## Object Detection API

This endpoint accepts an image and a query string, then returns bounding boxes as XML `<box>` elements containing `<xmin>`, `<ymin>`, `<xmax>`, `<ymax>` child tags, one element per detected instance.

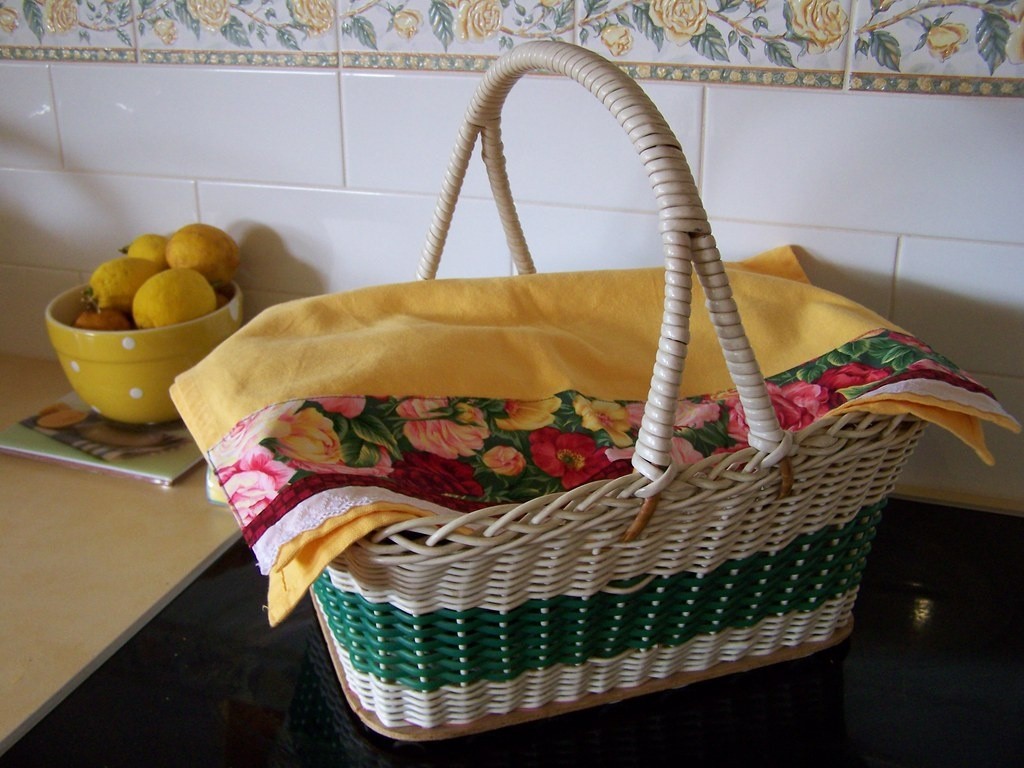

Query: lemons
<box><xmin>71</xmin><ymin>222</ymin><xmax>238</xmax><ymax>331</ymax></box>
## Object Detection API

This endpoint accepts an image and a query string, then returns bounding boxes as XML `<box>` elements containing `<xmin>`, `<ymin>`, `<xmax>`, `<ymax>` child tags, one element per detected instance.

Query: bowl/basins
<box><xmin>43</xmin><ymin>276</ymin><xmax>243</xmax><ymax>425</ymax></box>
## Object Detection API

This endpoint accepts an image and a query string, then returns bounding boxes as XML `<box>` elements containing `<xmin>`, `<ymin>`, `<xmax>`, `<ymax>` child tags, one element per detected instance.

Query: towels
<box><xmin>165</xmin><ymin>235</ymin><xmax>1024</xmax><ymax>629</ymax></box>
